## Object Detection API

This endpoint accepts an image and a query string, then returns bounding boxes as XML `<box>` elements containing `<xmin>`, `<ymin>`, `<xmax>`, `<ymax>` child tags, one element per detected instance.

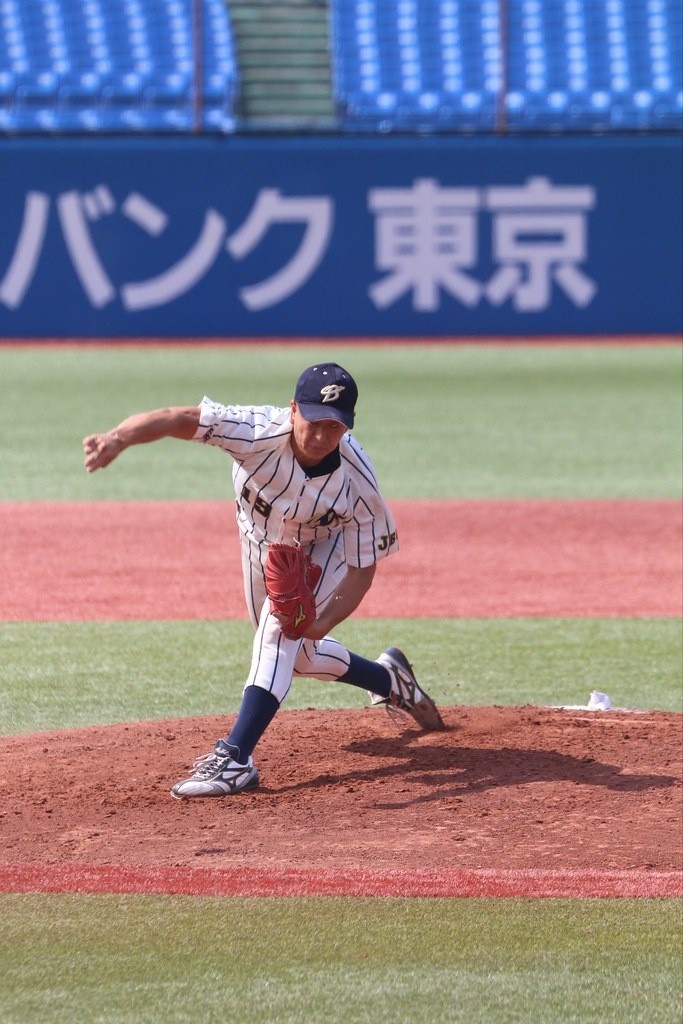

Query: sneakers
<box><xmin>367</xmin><ymin>647</ymin><xmax>444</xmax><ymax>732</ymax></box>
<box><xmin>170</xmin><ymin>738</ymin><xmax>259</xmax><ymax>800</ymax></box>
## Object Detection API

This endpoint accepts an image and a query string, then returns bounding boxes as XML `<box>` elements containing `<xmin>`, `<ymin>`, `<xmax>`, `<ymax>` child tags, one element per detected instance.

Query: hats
<box><xmin>294</xmin><ymin>363</ymin><xmax>358</xmax><ymax>430</ymax></box>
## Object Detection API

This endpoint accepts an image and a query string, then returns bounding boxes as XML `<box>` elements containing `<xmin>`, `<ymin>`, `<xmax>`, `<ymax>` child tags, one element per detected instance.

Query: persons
<box><xmin>83</xmin><ymin>362</ymin><xmax>444</xmax><ymax>799</ymax></box>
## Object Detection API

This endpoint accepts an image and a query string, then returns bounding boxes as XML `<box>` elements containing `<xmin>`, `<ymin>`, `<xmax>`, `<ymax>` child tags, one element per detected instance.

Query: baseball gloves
<box><xmin>262</xmin><ymin>539</ymin><xmax>324</xmax><ymax>642</ymax></box>
